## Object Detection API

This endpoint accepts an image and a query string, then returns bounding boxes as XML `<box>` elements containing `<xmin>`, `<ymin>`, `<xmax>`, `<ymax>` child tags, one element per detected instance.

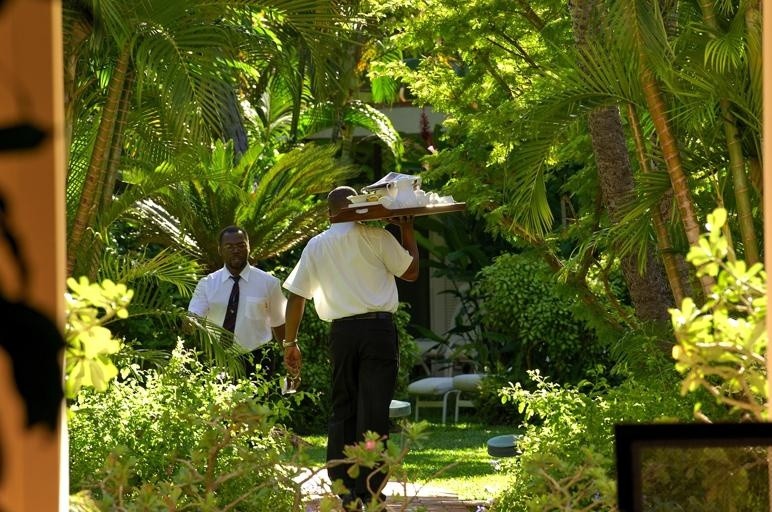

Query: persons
<box><xmin>280</xmin><ymin>184</ymin><xmax>422</xmax><ymax>512</ymax></box>
<box><xmin>186</xmin><ymin>223</ymin><xmax>288</xmax><ymax>381</ymax></box>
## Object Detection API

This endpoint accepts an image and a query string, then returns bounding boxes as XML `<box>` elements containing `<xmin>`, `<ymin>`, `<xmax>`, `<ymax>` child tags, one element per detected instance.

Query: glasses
<box><xmin>221</xmin><ymin>242</ymin><xmax>248</xmax><ymax>253</ymax></box>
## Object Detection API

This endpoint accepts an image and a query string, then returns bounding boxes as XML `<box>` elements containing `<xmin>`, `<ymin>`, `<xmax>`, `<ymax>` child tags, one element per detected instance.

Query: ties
<box><xmin>219</xmin><ymin>276</ymin><xmax>242</xmax><ymax>350</ymax></box>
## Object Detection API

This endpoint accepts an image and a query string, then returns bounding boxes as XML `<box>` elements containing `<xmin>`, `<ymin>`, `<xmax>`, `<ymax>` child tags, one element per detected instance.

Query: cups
<box><xmin>429</xmin><ymin>193</ymin><xmax>454</xmax><ymax>206</ymax></box>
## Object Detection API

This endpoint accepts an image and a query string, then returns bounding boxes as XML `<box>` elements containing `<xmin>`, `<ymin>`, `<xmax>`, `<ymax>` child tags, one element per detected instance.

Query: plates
<box><xmin>346</xmin><ymin>195</ymin><xmax>369</xmax><ymax>204</ymax></box>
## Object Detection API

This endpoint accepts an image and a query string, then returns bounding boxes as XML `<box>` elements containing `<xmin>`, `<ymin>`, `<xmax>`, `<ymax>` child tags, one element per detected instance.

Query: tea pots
<box><xmin>386</xmin><ymin>177</ymin><xmax>433</xmax><ymax>213</ymax></box>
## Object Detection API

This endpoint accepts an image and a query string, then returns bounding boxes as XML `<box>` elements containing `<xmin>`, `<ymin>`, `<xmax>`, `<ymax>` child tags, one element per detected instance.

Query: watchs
<box><xmin>282</xmin><ymin>337</ymin><xmax>299</xmax><ymax>350</ymax></box>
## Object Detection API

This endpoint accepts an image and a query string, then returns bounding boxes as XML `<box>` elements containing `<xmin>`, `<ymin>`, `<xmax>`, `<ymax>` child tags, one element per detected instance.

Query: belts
<box><xmin>331</xmin><ymin>311</ymin><xmax>394</xmax><ymax>321</ymax></box>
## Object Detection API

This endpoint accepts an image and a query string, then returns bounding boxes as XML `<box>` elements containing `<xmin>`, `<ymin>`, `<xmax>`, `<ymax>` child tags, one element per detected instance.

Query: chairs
<box><xmin>407</xmin><ymin>343</ymin><xmax>502</xmax><ymax>427</ymax></box>
